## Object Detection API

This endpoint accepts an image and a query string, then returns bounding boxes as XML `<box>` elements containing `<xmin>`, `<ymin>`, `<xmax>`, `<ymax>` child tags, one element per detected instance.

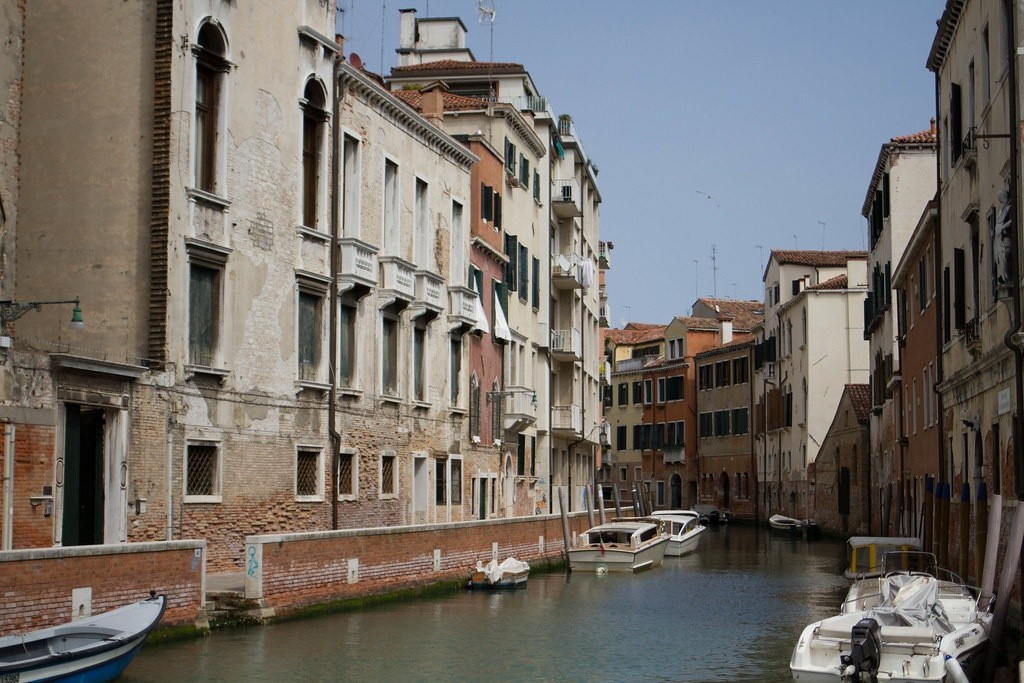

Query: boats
<box><xmin>645</xmin><ymin>510</ymin><xmax>707</xmax><ymax>557</ymax></box>
<box><xmin>2</xmin><ymin>590</ymin><xmax>171</xmax><ymax>683</ymax></box>
<box><xmin>471</xmin><ymin>557</ymin><xmax>531</xmax><ymax>588</ymax></box>
<box><xmin>790</xmin><ymin>536</ymin><xmax>992</xmax><ymax>683</ymax></box>
<box><xmin>566</xmin><ymin>516</ymin><xmax>673</xmax><ymax>576</ymax></box>
<box><xmin>769</xmin><ymin>513</ymin><xmax>803</xmax><ymax>532</ymax></box>
